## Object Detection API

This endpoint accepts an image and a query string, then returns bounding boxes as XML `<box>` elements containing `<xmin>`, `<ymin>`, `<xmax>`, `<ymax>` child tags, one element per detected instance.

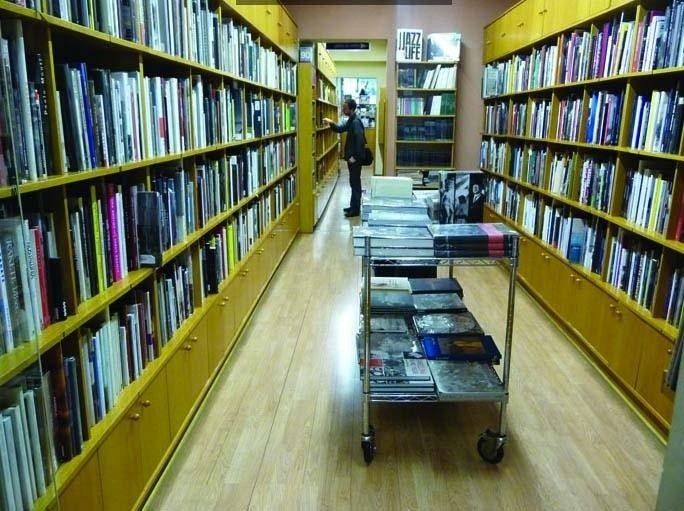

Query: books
<box><xmin>1</xmin><ymin>1</ymin><xmax>297</xmax><ymax>510</ymax></box>
<box><xmin>343</xmin><ymin>173</ymin><xmax>519</xmax><ymax>404</ymax></box>
<box><xmin>482</xmin><ymin>0</ymin><xmax>684</xmax><ymax>328</ymax></box>
<box><xmin>396</xmin><ymin>65</ymin><xmax>456</xmax><ymax>185</ymax></box>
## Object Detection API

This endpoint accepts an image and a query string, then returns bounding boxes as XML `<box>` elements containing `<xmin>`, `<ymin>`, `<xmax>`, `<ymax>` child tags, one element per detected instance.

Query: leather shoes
<box><xmin>344</xmin><ymin>207</ymin><xmax>359</xmax><ymax>217</ymax></box>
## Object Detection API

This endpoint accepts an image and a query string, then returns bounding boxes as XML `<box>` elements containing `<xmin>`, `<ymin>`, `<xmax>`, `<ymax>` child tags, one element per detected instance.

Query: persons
<box><xmin>322</xmin><ymin>99</ymin><xmax>365</xmax><ymax>218</ymax></box>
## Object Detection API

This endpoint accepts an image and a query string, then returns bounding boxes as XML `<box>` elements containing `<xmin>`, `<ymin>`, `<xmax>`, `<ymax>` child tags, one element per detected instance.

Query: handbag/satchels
<box><xmin>361</xmin><ymin>148</ymin><xmax>373</xmax><ymax>165</ymax></box>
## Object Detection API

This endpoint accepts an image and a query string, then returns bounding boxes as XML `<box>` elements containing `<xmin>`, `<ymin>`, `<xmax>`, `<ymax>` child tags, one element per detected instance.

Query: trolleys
<box><xmin>360</xmin><ymin>184</ymin><xmax>518</xmax><ymax>466</ymax></box>
